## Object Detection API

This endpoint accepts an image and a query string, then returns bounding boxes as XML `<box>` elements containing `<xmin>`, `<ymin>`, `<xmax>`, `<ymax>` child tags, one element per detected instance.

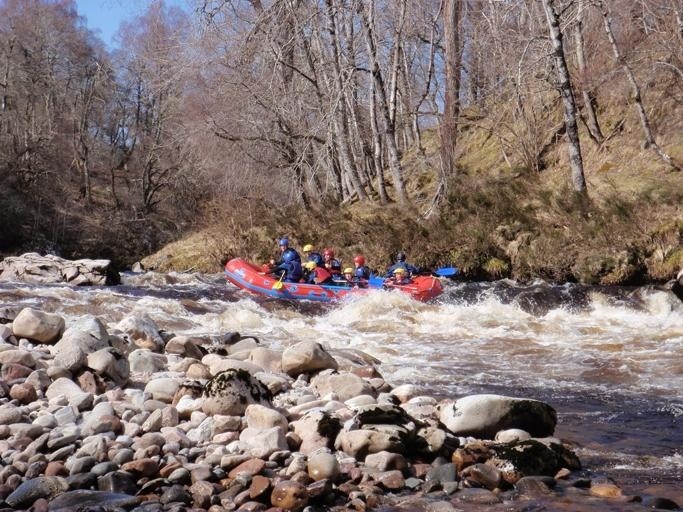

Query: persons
<box><xmin>300</xmin><ymin>243</ymin><xmax>370</xmax><ymax>292</ymax></box>
<box><xmin>262</xmin><ymin>237</ymin><xmax>301</xmax><ymax>269</ymax></box>
<box><xmin>254</xmin><ymin>248</ymin><xmax>302</xmax><ymax>283</ymax></box>
<box><xmin>383</xmin><ymin>268</ymin><xmax>414</xmax><ymax>288</ymax></box>
<box><xmin>384</xmin><ymin>252</ymin><xmax>420</xmax><ymax>279</ymax></box>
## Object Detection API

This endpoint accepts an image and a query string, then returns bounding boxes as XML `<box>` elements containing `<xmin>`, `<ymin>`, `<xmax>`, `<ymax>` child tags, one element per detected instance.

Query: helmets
<box><xmin>394</xmin><ymin>268</ymin><xmax>404</xmax><ymax>276</ymax></box>
<box><xmin>353</xmin><ymin>255</ymin><xmax>365</xmax><ymax>266</ymax></box>
<box><xmin>343</xmin><ymin>268</ymin><xmax>355</xmax><ymax>277</ymax></box>
<box><xmin>278</xmin><ymin>239</ymin><xmax>289</xmax><ymax>248</ymax></box>
<box><xmin>397</xmin><ymin>253</ymin><xmax>406</xmax><ymax>261</ymax></box>
<box><xmin>305</xmin><ymin>261</ymin><xmax>317</xmax><ymax>272</ymax></box>
<box><xmin>302</xmin><ymin>244</ymin><xmax>316</xmax><ymax>253</ymax></box>
<box><xmin>323</xmin><ymin>249</ymin><xmax>335</xmax><ymax>259</ymax></box>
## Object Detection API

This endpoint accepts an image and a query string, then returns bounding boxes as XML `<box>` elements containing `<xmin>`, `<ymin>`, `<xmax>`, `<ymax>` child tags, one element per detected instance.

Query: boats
<box><xmin>224</xmin><ymin>257</ymin><xmax>444</xmax><ymax>305</ymax></box>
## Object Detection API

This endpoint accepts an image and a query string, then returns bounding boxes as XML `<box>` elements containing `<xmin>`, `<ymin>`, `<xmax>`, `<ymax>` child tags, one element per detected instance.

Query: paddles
<box><xmin>332</xmin><ymin>278</ymin><xmax>384</xmax><ymax>288</ymax></box>
<box><xmin>369</xmin><ymin>270</ymin><xmax>374</xmax><ymax>278</ymax></box>
<box><xmin>412</xmin><ymin>268</ymin><xmax>458</xmax><ymax>275</ymax></box>
<box><xmin>271</xmin><ymin>270</ymin><xmax>285</xmax><ymax>289</ymax></box>
